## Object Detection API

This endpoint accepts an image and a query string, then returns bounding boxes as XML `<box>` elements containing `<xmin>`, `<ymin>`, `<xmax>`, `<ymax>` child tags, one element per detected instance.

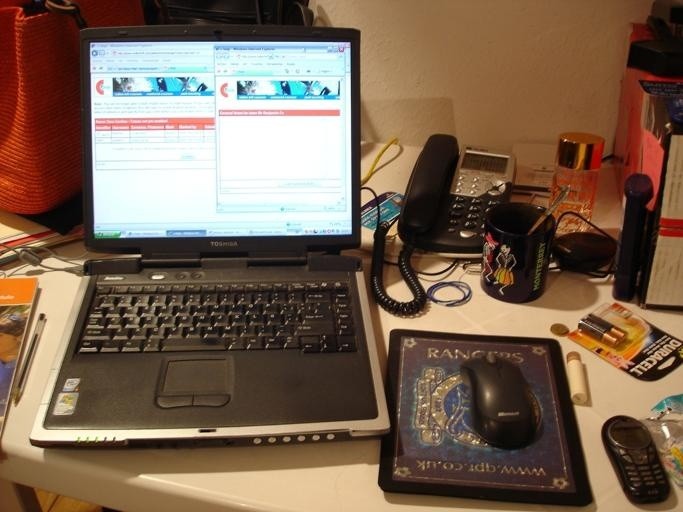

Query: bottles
<box><xmin>550</xmin><ymin>132</ymin><xmax>606</xmax><ymax>234</ymax></box>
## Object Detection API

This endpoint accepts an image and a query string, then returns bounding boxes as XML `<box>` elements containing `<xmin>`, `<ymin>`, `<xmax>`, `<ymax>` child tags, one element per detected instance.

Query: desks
<box><xmin>0</xmin><ymin>141</ymin><xmax>683</xmax><ymax>511</ymax></box>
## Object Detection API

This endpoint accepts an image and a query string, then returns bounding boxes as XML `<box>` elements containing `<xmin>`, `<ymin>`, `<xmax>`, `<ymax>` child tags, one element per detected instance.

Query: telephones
<box><xmin>370</xmin><ymin>131</ymin><xmax>517</xmax><ymax>315</ymax></box>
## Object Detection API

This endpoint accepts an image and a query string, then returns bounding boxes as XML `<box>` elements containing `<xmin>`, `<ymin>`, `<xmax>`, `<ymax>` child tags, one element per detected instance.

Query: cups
<box><xmin>480</xmin><ymin>202</ymin><xmax>556</xmax><ymax>303</ymax></box>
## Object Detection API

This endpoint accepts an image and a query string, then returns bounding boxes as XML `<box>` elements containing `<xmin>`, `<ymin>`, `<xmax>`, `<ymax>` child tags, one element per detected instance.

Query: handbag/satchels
<box><xmin>0</xmin><ymin>0</ymin><xmax>148</xmax><ymax>217</ymax></box>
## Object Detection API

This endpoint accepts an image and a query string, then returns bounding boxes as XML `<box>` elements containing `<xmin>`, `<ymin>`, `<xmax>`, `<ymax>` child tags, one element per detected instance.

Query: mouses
<box><xmin>459</xmin><ymin>352</ymin><xmax>535</xmax><ymax>450</ymax></box>
<box><xmin>553</xmin><ymin>232</ymin><xmax>616</xmax><ymax>273</ymax></box>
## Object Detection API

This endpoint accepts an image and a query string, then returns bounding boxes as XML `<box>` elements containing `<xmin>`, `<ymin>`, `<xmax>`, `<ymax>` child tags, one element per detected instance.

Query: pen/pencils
<box><xmin>528</xmin><ymin>184</ymin><xmax>571</xmax><ymax>234</ymax></box>
<box><xmin>13</xmin><ymin>312</ymin><xmax>45</xmax><ymax>407</ymax></box>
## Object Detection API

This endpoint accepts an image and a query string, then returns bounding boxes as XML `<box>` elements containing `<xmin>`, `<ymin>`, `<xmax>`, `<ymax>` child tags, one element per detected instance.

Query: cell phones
<box><xmin>601</xmin><ymin>415</ymin><xmax>671</xmax><ymax>505</ymax></box>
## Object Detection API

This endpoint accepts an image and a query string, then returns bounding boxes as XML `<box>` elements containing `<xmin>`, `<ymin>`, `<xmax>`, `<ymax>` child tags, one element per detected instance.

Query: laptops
<box><xmin>27</xmin><ymin>25</ymin><xmax>392</xmax><ymax>451</ymax></box>
